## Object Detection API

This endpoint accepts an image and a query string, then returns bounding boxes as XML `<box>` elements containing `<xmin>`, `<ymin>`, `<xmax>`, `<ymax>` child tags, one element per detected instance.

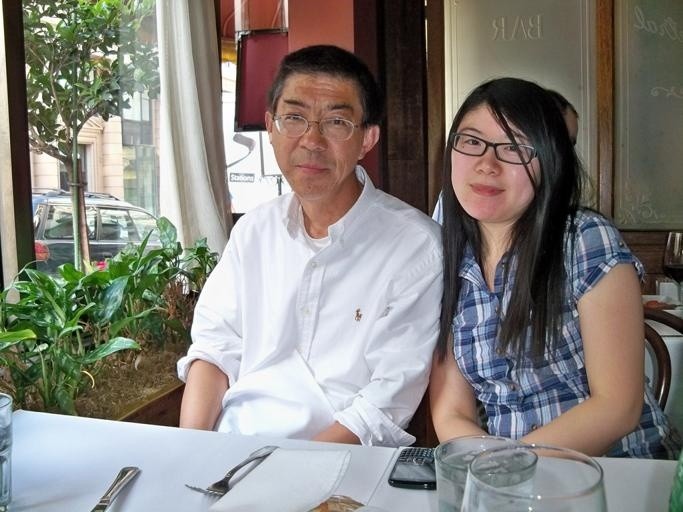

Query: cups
<box><xmin>0</xmin><ymin>393</ymin><xmax>14</xmax><ymax>512</ymax></box>
<box><xmin>434</xmin><ymin>434</ymin><xmax>607</xmax><ymax>512</ymax></box>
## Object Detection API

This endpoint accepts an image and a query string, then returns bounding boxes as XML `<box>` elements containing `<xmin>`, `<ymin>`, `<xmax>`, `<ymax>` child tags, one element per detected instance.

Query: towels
<box><xmin>209</xmin><ymin>447</ymin><xmax>353</xmax><ymax>511</ymax></box>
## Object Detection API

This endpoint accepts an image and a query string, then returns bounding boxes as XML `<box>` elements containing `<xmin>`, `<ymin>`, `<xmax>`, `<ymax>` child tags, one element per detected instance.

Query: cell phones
<box><xmin>388</xmin><ymin>447</ymin><xmax>436</xmax><ymax>490</ymax></box>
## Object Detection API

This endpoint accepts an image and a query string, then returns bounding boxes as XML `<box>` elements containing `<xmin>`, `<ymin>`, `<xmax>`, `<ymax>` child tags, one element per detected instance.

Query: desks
<box><xmin>641</xmin><ymin>290</ymin><xmax>682</xmax><ymax>433</ymax></box>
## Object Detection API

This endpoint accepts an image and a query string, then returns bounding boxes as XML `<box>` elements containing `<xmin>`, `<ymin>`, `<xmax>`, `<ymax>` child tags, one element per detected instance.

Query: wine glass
<box><xmin>662</xmin><ymin>231</ymin><xmax>683</xmax><ymax>310</ymax></box>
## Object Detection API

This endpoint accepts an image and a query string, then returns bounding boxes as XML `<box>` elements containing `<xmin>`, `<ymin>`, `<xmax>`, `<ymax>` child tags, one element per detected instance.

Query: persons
<box><xmin>427</xmin><ymin>77</ymin><xmax>681</xmax><ymax>462</ymax></box>
<box><xmin>174</xmin><ymin>43</ymin><xmax>446</xmax><ymax>447</ymax></box>
<box><xmin>431</xmin><ymin>90</ymin><xmax>579</xmax><ymax>226</ymax></box>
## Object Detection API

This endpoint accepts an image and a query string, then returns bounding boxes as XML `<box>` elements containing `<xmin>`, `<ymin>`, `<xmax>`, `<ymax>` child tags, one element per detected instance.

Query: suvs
<box><xmin>31</xmin><ymin>185</ymin><xmax>169</xmax><ymax>279</ymax></box>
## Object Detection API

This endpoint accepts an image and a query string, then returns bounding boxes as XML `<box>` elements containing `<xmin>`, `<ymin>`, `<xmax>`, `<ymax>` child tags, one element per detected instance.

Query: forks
<box><xmin>183</xmin><ymin>443</ymin><xmax>281</xmax><ymax>499</ymax></box>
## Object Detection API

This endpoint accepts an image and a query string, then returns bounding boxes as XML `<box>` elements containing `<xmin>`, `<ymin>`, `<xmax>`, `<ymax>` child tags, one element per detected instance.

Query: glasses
<box><xmin>448</xmin><ymin>132</ymin><xmax>542</xmax><ymax>165</ymax></box>
<box><xmin>272</xmin><ymin>114</ymin><xmax>368</xmax><ymax>141</ymax></box>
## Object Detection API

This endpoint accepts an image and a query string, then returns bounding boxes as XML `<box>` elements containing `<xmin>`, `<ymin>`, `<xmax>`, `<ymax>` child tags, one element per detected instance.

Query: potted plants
<box><xmin>0</xmin><ymin>217</ymin><xmax>219</xmax><ymax>410</ymax></box>
